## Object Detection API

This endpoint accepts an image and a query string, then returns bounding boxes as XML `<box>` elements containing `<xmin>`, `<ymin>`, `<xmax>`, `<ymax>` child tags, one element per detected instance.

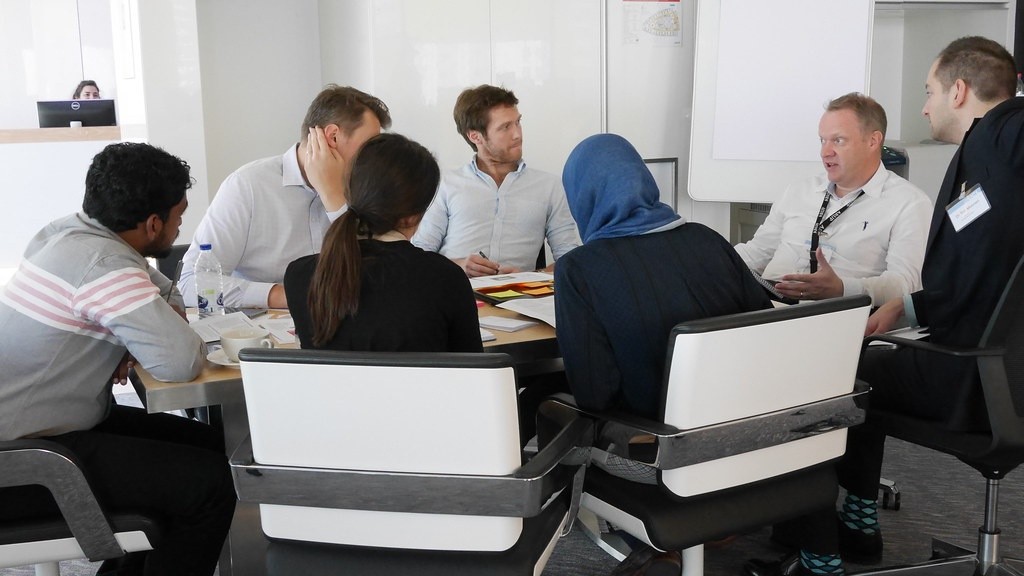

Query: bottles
<box><xmin>194</xmin><ymin>243</ymin><xmax>225</xmax><ymax>320</ymax></box>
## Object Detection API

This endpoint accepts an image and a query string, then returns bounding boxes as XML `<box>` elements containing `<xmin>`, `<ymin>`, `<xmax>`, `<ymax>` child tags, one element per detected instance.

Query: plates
<box><xmin>206</xmin><ymin>346</ymin><xmax>279</xmax><ymax>369</ymax></box>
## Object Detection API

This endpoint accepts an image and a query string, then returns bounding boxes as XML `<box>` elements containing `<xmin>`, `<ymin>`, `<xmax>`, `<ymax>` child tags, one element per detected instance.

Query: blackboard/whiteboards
<box><xmin>687</xmin><ymin>0</ymin><xmax>874</xmax><ymax>204</ymax></box>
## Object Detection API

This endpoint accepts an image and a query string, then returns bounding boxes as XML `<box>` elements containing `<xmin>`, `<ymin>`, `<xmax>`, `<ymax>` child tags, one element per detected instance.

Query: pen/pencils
<box><xmin>167</xmin><ymin>260</ymin><xmax>184</xmax><ymax>305</ymax></box>
<box><xmin>479</xmin><ymin>251</ymin><xmax>499</xmax><ymax>272</ymax></box>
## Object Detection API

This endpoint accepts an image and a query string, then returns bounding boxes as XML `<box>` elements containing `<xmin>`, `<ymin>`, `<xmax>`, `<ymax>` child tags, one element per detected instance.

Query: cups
<box><xmin>220</xmin><ymin>328</ymin><xmax>275</xmax><ymax>363</ymax></box>
<box><xmin>70</xmin><ymin>121</ymin><xmax>82</xmax><ymax>127</ymax></box>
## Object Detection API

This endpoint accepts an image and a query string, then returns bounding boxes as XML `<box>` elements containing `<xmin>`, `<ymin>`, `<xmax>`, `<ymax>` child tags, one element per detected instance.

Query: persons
<box><xmin>553</xmin><ymin>133</ymin><xmax>774</xmax><ymax>576</ymax></box>
<box><xmin>73</xmin><ymin>80</ymin><xmax>99</xmax><ymax>100</ymax></box>
<box><xmin>410</xmin><ymin>84</ymin><xmax>579</xmax><ymax>279</ymax></box>
<box><xmin>734</xmin><ymin>92</ymin><xmax>935</xmax><ymax>306</ymax></box>
<box><xmin>0</xmin><ymin>143</ymin><xmax>236</xmax><ymax>576</ymax></box>
<box><xmin>743</xmin><ymin>35</ymin><xmax>1024</xmax><ymax>575</ymax></box>
<box><xmin>283</xmin><ymin>132</ymin><xmax>558</xmax><ymax>451</ymax></box>
<box><xmin>175</xmin><ymin>86</ymin><xmax>391</xmax><ymax>308</ymax></box>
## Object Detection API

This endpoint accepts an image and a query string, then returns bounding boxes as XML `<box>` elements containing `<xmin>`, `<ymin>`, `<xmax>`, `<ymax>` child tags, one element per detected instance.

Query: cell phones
<box><xmin>236</xmin><ymin>307</ymin><xmax>268</xmax><ymax>319</ymax></box>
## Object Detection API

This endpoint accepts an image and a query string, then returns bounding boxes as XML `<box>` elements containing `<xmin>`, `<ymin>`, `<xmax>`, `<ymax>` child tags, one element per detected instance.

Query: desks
<box><xmin>128</xmin><ymin>270</ymin><xmax>555</xmax><ymax>576</ymax></box>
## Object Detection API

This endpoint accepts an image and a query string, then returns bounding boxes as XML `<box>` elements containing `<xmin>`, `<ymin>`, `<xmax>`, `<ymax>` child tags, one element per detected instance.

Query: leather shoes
<box><xmin>838</xmin><ymin>517</ymin><xmax>883</xmax><ymax>566</ymax></box>
<box><xmin>744</xmin><ymin>553</ymin><xmax>817</xmax><ymax>576</ymax></box>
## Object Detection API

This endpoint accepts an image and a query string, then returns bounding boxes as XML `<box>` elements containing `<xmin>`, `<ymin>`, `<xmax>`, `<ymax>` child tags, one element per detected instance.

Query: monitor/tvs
<box><xmin>37</xmin><ymin>99</ymin><xmax>117</xmax><ymax>127</ymax></box>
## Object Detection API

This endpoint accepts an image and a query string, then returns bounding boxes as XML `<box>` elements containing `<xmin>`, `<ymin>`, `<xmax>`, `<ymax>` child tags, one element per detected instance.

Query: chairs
<box><xmin>536</xmin><ymin>294</ymin><xmax>872</xmax><ymax>576</ymax></box>
<box><xmin>0</xmin><ymin>438</ymin><xmax>160</xmax><ymax>576</ymax></box>
<box><xmin>851</xmin><ymin>254</ymin><xmax>1024</xmax><ymax>576</ymax></box>
<box><xmin>229</xmin><ymin>347</ymin><xmax>597</xmax><ymax>575</ymax></box>
